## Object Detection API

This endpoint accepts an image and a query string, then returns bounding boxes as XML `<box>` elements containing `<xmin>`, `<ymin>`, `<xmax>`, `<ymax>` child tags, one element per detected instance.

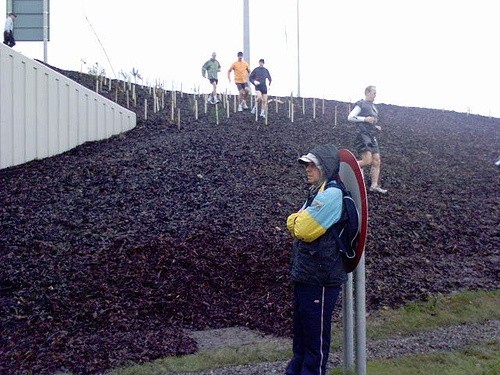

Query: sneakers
<box><xmin>369</xmin><ymin>185</ymin><xmax>388</xmax><ymax>194</ymax></box>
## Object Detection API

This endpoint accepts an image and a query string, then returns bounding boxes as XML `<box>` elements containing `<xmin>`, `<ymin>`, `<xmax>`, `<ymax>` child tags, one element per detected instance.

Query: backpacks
<box><xmin>323</xmin><ymin>182</ymin><xmax>362</xmax><ymax>263</ymax></box>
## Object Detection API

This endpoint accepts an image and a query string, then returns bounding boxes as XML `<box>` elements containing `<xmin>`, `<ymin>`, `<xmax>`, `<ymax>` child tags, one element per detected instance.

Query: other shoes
<box><xmin>214</xmin><ymin>99</ymin><xmax>220</xmax><ymax>105</ymax></box>
<box><xmin>260</xmin><ymin>113</ymin><xmax>266</xmax><ymax>118</ymax></box>
<box><xmin>237</xmin><ymin>104</ymin><xmax>243</xmax><ymax>112</ymax></box>
<box><xmin>251</xmin><ymin>106</ymin><xmax>256</xmax><ymax>114</ymax></box>
<box><xmin>242</xmin><ymin>104</ymin><xmax>249</xmax><ymax>109</ymax></box>
<box><xmin>208</xmin><ymin>98</ymin><xmax>214</xmax><ymax>105</ymax></box>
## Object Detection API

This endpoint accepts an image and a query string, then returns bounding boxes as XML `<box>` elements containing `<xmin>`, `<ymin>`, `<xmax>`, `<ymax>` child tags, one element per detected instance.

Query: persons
<box><xmin>348</xmin><ymin>85</ymin><xmax>387</xmax><ymax>194</ymax></box>
<box><xmin>3</xmin><ymin>13</ymin><xmax>16</xmax><ymax>47</ymax></box>
<box><xmin>202</xmin><ymin>53</ymin><xmax>221</xmax><ymax>105</ymax></box>
<box><xmin>249</xmin><ymin>58</ymin><xmax>272</xmax><ymax>117</ymax></box>
<box><xmin>284</xmin><ymin>143</ymin><xmax>348</xmax><ymax>375</ymax></box>
<box><xmin>227</xmin><ymin>51</ymin><xmax>251</xmax><ymax>112</ymax></box>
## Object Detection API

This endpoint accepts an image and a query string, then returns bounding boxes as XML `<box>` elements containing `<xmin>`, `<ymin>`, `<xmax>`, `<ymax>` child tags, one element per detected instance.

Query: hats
<box><xmin>297</xmin><ymin>156</ymin><xmax>313</xmax><ymax>165</ymax></box>
